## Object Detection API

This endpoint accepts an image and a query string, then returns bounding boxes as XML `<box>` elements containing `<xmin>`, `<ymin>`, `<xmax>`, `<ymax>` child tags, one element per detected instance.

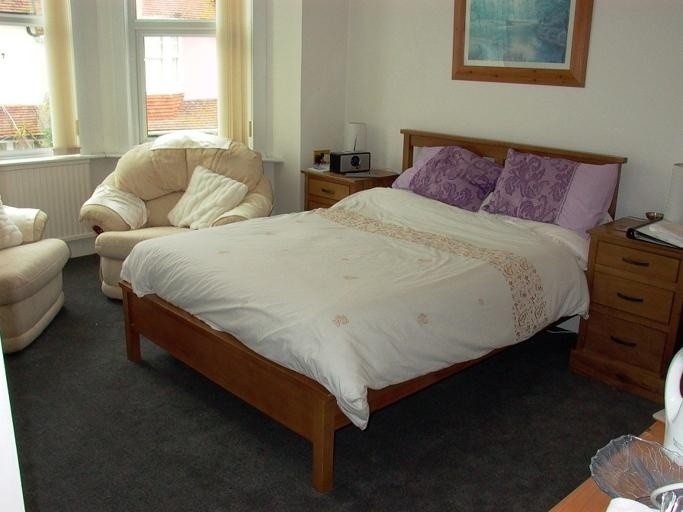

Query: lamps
<box><xmin>665</xmin><ymin>162</ymin><xmax>683</xmax><ymax>225</ymax></box>
<box><xmin>344</xmin><ymin>122</ymin><xmax>366</xmax><ymax>154</ymax></box>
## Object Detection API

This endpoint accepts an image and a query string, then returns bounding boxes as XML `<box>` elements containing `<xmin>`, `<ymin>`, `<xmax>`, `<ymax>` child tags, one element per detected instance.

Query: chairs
<box><xmin>79</xmin><ymin>139</ymin><xmax>274</xmax><ymax>300</ymax></box>
<box><xmin>0</xmin><ymin>198</ymin><xmax>71</xmax><ymax>354</ymax></box>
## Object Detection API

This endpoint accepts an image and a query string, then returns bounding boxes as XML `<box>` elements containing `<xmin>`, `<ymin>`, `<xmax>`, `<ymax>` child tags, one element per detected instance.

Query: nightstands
<box><xmin>571</xmin><ymin>217</ymin><xmax>683</xmax><ymax>405</ymax></box>
<box><xmin>302</xmin><ymin>168</ymin><xmax>400</xmax><ymax>209</ymax></box>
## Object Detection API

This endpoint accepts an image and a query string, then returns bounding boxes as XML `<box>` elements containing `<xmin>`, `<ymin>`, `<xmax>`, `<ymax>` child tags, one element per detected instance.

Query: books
<box><xmin>626</xmin><ymin>218</ymin><xmax>683</xmax><ymax>249</ymax></box>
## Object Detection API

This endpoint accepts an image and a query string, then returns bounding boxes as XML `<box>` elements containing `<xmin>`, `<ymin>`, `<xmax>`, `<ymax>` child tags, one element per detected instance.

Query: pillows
<box><xmin>166</xmin><ymin>168</ymin><xmax>247</xmax><ymax>231</ymax></box>
<box><xmin>392</xmin><ymin>144</ymin><xmax>500</xmax><ymax>212</ymax></box>
<box><xmin>0</xmin><ymin>209</ymin><xmax>22</xmax><ymax>250</ymax></box>
<box><xmin>483</xmin><ymin>149</ymin><xmax>619</xmax><ymax>241</ymax></box>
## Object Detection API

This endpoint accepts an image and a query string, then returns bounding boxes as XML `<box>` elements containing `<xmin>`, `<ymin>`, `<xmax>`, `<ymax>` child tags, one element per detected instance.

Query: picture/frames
<box><xmin>450</xmin><ymin>0</ymin><xmax>593</xmax><ymax>88</ymax></box>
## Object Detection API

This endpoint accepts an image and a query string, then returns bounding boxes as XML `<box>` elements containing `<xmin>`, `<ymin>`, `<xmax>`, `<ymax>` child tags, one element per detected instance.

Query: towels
<box><xmin>151</xmin><ymin>128</ymin><xmax>232</xmax><ymax>152</ymax></box>
<box><xmin>83</xmin><ymin>186</ymin><xmax>147</xmax><ymax>228</ymax></box>
<box><xmin>213</xmin><ymin>192</ymin><xmax>270</xmax><ymax>221</ymax></box>
<box><xmin>0</xmin><ymin>202</ymin><xmax>38</xmax><ymax>241</ymax></box>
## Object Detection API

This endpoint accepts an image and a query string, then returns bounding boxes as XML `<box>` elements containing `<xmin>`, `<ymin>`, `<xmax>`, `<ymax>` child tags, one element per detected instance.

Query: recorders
<box><xmin>330</xmin><ymin>136</ymin><xmax>371</xmax><ymax>173</ymax></box>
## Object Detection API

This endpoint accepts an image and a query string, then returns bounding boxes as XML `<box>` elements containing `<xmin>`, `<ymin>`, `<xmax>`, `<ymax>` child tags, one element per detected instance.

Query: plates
<box><xmin>588</xmin><ymin>434</ymin><xmax>683</xmax><ymax>512</ymax></box>
<box><xmin>646</xmin><ymin>212</ymin><xmax>664</xmax><ymax>221</ymax></box>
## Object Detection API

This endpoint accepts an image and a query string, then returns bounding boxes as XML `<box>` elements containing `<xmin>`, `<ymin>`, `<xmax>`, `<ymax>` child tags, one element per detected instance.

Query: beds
<box><xmin>114</xmin><ymin>127</ymin><xmax>628</xmax><ymax>495</ymax></box>
